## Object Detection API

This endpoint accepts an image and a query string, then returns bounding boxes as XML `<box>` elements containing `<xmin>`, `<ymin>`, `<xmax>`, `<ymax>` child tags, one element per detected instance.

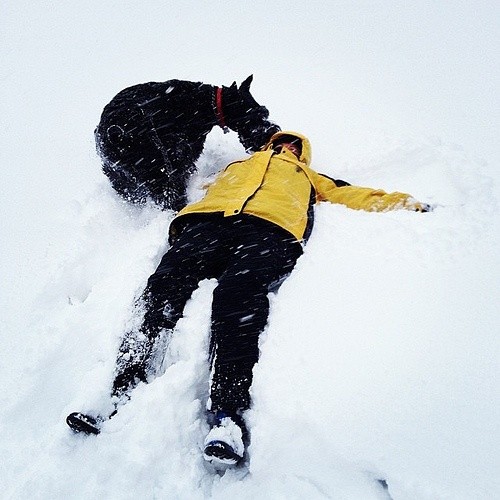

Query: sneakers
<box><xmin>67</xmin><ymin>412</ymin><xmax>102</xmax><ymax>435</ymax></box>
<box><xmin>205</xmin><ymin>413</ymin><xmax>248</xmax><ymax>465</ymax></box>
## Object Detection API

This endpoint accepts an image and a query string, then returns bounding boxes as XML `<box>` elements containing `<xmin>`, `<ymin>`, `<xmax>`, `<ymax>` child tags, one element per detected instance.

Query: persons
<box><xmin>65</xmin><ymin>130</ymin><xmax>434</xmax><ymax>471</ymax></box>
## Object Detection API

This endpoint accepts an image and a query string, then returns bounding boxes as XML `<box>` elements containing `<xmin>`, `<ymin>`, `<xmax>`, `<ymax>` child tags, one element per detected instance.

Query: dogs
<box><xmin>93</xmin><ymin>74</ymin><xmax>282</xmax><ymax>215</ymax></box>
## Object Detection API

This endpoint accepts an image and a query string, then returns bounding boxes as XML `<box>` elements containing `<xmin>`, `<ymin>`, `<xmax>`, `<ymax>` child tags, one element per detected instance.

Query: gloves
<box><xmin>404</xmin><ymin>198</ymin><xmax>431</xmax><ymax>214</ymax></box>
